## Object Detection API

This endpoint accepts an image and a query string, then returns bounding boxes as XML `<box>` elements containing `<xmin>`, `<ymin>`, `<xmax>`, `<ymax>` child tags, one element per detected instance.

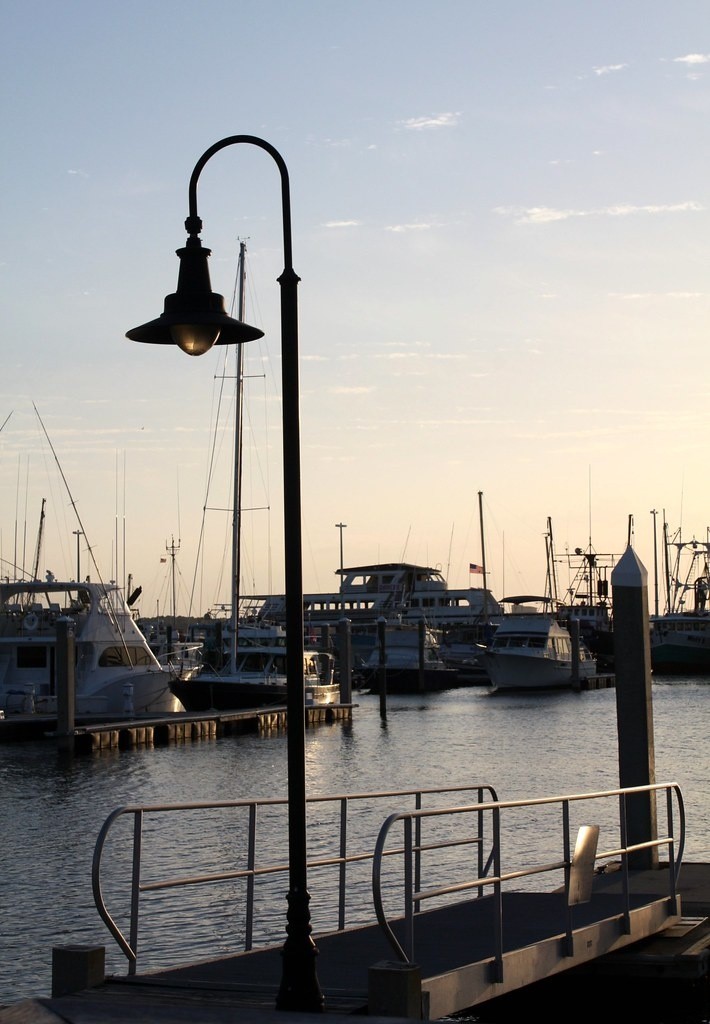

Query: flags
<box><xmin>309</xmin><ymin>622</ymin><xmax>317</xmax><ymax>644</ymax></box>
<box><xmin>469</xmin><ymin>563</ymin><xmax>483</xmax><ymax>574</ymax></box>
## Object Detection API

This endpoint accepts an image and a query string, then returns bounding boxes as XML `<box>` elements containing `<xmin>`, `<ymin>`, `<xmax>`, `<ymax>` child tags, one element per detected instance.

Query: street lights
<box><xmin>125</xmin><ymin>134</ymin><xmax>324</xmax><ymax>1013</ymax></box>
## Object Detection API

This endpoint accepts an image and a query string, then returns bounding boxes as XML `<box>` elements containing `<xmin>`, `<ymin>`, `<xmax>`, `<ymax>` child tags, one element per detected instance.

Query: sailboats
<box><xmin>167</xmin><ymin>236</ymin><xmax>338</xmax><ymax>737</ymax></box>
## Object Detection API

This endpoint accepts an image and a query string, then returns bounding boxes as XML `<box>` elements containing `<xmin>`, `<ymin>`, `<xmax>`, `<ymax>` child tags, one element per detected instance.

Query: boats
<box><xmin>128</xmin><ymin>533</ymin><xmax>206</xmax><ymax>688</ymax></box>
<box><xmin>646</xmin><ymin>507</ymin><xmax>710</xmax><ymax>677</ymax></box>
<box><xmin>238</xmin><ymin>522</ymin><xmax>504</xmax><ymax>695</ymax></box>
<box><xmin>0</xmin><ymin>397</ymin><xmax>189</xmax><ymax>742</ymax></box>
<box><xmin>475</xmin><ymin>516</ymin><xmax>615</xmax><ymax>695</ymax></box>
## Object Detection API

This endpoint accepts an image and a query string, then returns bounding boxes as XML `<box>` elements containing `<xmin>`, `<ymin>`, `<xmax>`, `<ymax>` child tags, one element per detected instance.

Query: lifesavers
<box><xmin>23</xmin><ymin>613</ymin><xmax>38</xmax><ymax>631</ymax></box>
